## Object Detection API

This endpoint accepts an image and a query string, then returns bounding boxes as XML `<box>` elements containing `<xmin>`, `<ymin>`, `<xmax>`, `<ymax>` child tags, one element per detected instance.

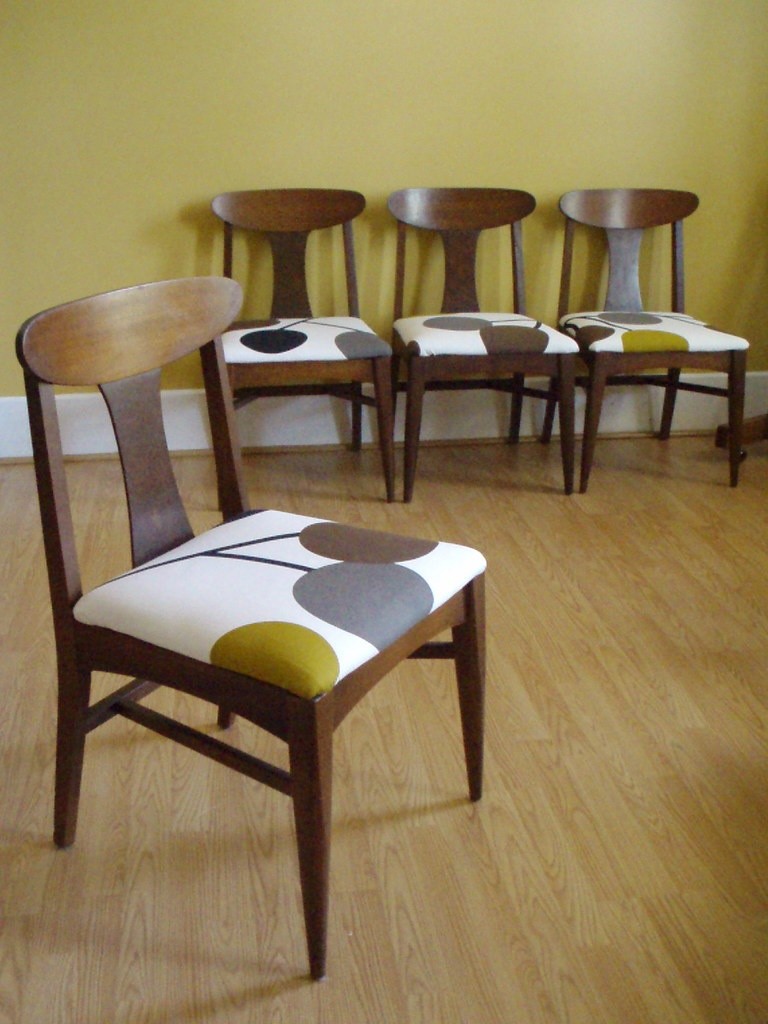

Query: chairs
<box><xmin>12</xmin><ymin>277</ymin><xmax>489</xmax><ymax>979</ymax></box>
<box><xmin>209</xmin><ymin>190</ymin><xmax>396</xmax><ymax>506</ymax></box>
<box><xmin>541</xmin><ymin>189</ymin><xmax>748</xmax><ymax>496</ymax></box>
<box><xmin>386</xmin><ymin>187</ymin><xmax>580</xmax><ymax>502</ymax></box>
